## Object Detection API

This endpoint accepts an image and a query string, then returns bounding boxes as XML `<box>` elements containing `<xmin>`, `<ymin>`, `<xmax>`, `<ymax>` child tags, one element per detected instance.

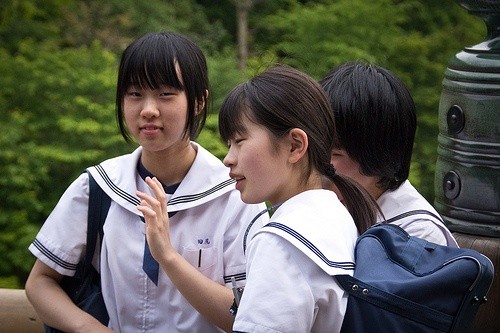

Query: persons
<box><xmin>218</xmin><ymin>64</ymin><xmax>390</xmax><ymax>333</ymax></box>
<box><xmin>318</xmin><ymin>59</ymin><xmax>460</xmax><ymax>252</ymax></box>
<box><xmin>24</xmin><ymin>29</ymin><xmax>270</xmax><ymax>333</ymax></box>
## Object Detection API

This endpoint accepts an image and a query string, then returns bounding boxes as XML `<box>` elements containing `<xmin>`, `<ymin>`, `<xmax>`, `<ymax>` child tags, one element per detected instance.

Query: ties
<box><xmin>137</xmin><ymin>209</ymin><xmax>179</xmax><ymax>287</ymax></box>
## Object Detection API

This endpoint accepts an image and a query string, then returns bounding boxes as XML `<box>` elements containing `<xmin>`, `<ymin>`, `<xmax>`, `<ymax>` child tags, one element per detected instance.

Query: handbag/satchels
<box><xmin>334</xmin><ymin>222</ymin><xmax>496</xmax><ymax>333</ymax></box>
<box><xmin>40</xmin><ymin>169</ymin><xmax>113</xmax><ymax>332</ymax></box>
